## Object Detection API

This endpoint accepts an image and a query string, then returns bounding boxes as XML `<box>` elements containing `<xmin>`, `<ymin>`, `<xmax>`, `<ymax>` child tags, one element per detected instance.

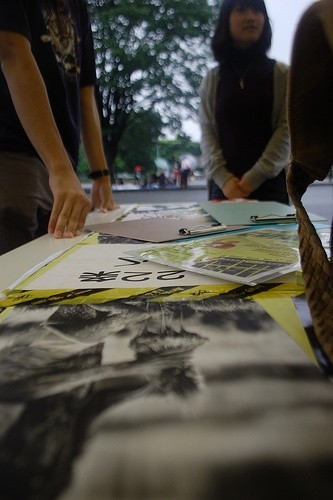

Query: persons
<box><xmin>0</xmin><ymin>0</ymin><xmax>121</xmax><ymax>256</ymax></box>
<box><xmin>199</xmin><ymin>0</ymin><xmax>290</xmax><ymax>206</ymax></box>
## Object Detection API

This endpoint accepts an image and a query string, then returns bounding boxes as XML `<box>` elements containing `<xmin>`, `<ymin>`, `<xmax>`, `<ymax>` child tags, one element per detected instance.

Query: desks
<box><xmin>0</xmin><ymin>202</ymin><xmax>333</xmax><ymax>330</ymax></box>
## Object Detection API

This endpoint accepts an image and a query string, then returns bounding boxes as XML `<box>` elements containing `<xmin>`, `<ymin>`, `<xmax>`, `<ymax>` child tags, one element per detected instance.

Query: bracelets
<box><xmin>88</xmin><ymin>169</ymin><xmax>110</xmax><ymax>179</ymax></box>
